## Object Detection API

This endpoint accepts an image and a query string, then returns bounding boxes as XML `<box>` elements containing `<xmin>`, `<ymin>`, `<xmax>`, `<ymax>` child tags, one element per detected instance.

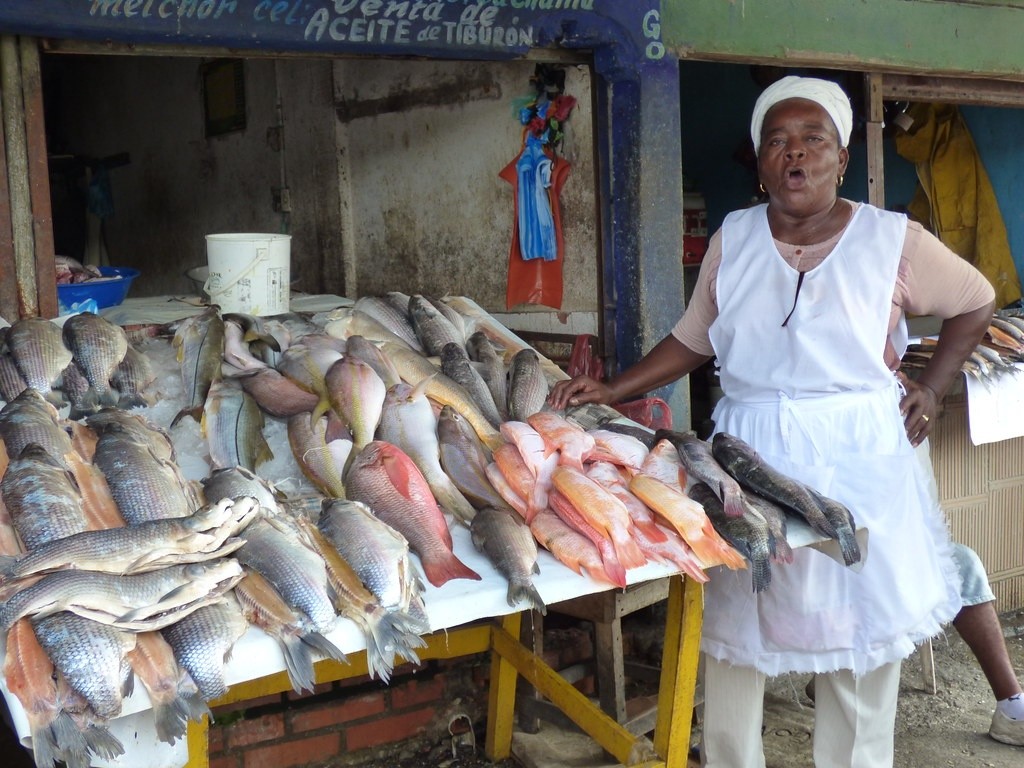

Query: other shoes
<box><xmin>987</xmin><ymin>708</ymin><xmax>1024</xmax><ymax>746</ymax></box>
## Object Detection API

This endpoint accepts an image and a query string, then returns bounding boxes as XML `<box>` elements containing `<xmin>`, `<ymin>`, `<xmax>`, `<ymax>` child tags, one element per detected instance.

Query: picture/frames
<box><xmin>199</xmin><ymin>58</ymin><xmax>246</xmax><ymax>138</ymax></box>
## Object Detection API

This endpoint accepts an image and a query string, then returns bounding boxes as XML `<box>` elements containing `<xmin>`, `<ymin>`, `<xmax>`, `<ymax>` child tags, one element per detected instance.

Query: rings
<box><xmin>922</xmin><ymin>415</ymin><xmax>929</xmax><ymax>421</ymax></box>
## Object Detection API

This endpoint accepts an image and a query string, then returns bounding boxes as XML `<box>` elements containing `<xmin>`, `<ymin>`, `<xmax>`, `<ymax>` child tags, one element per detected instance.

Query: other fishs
<box><xmin>0</xmin><ymin>289</ymin><xmax>863</xmax><ymax>768</ymax></box>
<box><xmin>902</xmin><ymin>317</ymin><xmax>1024</xmax><ymax>393</ymax></box>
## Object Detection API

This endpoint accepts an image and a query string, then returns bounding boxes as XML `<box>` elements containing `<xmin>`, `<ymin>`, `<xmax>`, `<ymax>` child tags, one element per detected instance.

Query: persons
<box><xmin>549</xmin><ymin>75</ymin><xmax>997</xmax><ymax>768</ymax></box>
<box><xmin>806</xmin><ymin>544</ymin><xmax>1024</xmax><ymax>746</ymax></box>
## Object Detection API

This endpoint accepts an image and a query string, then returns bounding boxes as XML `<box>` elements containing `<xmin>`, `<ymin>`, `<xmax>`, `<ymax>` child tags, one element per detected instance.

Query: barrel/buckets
<box><xmin>203</xmin><ymin>232</ymin><xmax>293</xmax><ymax>315</ymax></box>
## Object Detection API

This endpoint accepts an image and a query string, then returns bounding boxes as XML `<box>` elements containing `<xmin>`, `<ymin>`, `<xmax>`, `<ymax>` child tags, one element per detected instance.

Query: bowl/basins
<box><xmin>56</xmin><ymin>266</ymin><xmax>142</xmax><ymax>316</ymax></box>
<box><xmin>185</xmin><ymin>264</ymin><xmax>210</xmax><ymax>298</ymax></box>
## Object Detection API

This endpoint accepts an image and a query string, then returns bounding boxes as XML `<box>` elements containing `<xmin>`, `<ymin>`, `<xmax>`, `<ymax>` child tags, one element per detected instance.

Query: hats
<box><xmin>750</xmin><ymin>76</ymin><xmax>852</xmax><ymax>158</ymax></box>
